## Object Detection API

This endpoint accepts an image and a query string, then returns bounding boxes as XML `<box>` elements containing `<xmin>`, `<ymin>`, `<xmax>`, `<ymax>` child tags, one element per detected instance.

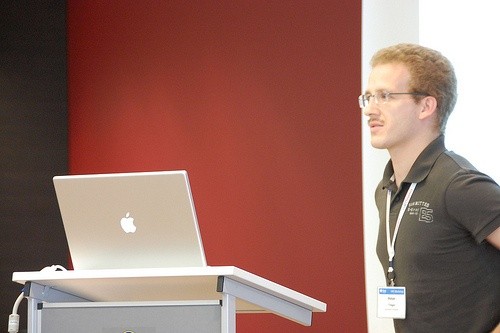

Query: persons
<box><xmin>362</xmin><ymin>43</ymin><xmax>500</xmax><ymax>333</ymax></box>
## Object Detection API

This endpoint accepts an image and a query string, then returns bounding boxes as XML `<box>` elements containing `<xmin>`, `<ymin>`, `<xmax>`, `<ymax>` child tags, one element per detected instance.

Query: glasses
<box><xmin>358</xmin><ymin>90</ymin><xmax>432</xmax><ymax>108</ymax></box>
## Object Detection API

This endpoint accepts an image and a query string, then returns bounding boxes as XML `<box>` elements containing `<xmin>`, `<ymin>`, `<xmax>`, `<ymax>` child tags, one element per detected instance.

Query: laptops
<box><xmin>52</xmin><ymin>170</ymin><xmax>214</xmax><ymax>270</ymax></box>
